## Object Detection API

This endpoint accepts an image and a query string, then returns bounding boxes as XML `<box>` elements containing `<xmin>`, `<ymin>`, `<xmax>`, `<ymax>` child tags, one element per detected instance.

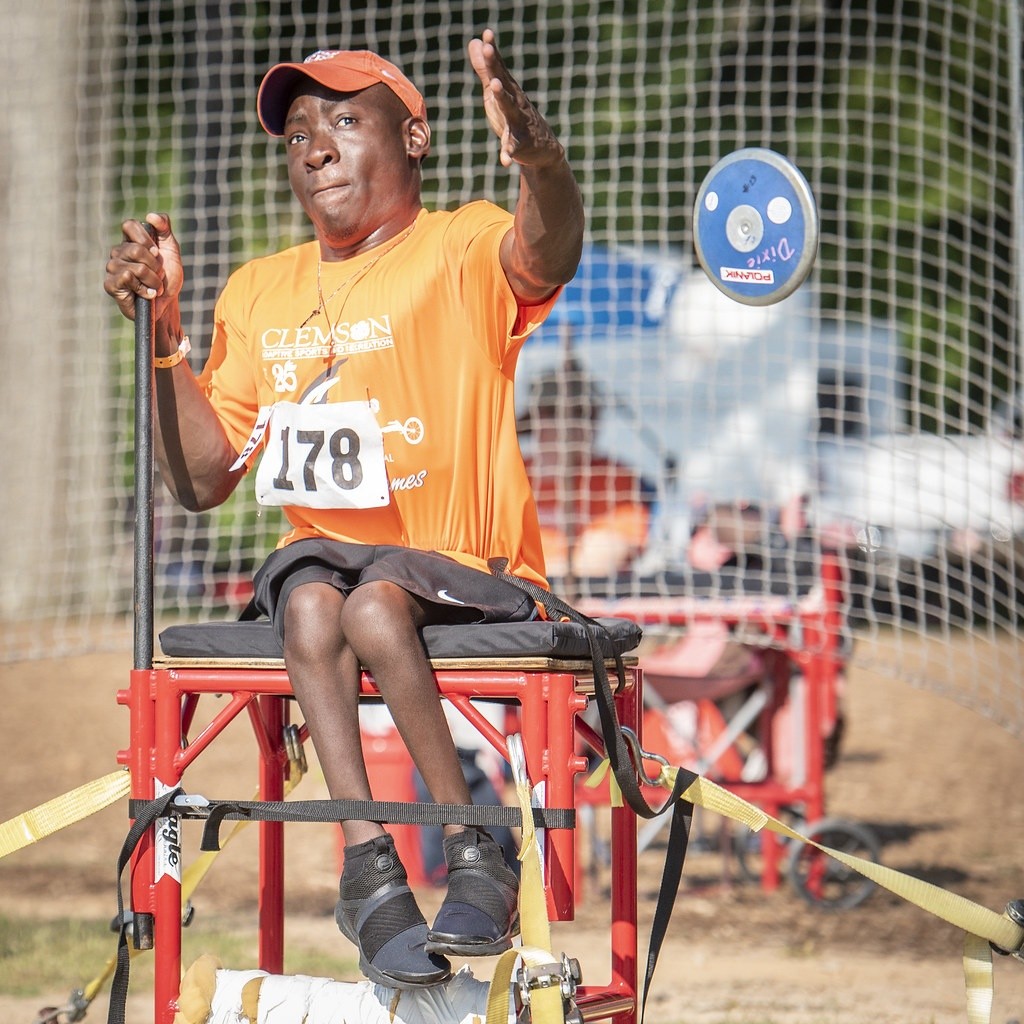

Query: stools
<box><xmin>117</xmin><ymin>609</ymin><xmax>643</xmax><ymax>1024</ymax></box>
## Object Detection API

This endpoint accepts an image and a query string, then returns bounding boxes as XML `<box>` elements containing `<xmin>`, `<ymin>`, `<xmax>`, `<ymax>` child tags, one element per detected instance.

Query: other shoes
<box><xmin>334</xmin><ymin>835</ymin><xmax>452</xmax><ymax>990</ymax></box>
<box><xmin>425</xmin><ymin>828</ymin><xmax>521</xmax><ymax>955</ymax></box>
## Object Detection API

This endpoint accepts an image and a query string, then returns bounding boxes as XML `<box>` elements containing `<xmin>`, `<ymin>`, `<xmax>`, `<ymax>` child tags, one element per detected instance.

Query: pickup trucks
<box><xmin>813</xmin><ymin>322</ymin><xmax>1024</xmax><ymax>605</ymax></box>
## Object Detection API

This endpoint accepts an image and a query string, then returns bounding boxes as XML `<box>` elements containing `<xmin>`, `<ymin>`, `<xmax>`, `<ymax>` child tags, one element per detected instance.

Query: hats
<box><xmin>513</xmin><ymin>360</ymin><xmax>607</xmax><ymax>438</ymax></box>
<box><xmin>257</xmin><ymin>49</ymin><xmax>428</xmax><ymax>165</ymax></box>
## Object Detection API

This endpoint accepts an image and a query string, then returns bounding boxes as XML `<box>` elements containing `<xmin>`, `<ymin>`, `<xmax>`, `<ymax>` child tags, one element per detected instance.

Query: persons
<box><xmin>515</xmin><ymin>355</ymin><xmax>812</xmax><ymax>579</ymax></box>
<box><xmin>103</xmin><ymin>27</ymin><xmax>586</xmax><ymax>991</ymax></box>
<box><xmin>152</xmin><ymin>333</ymin><xmax>193</xmax><ymax>369</ymax></box>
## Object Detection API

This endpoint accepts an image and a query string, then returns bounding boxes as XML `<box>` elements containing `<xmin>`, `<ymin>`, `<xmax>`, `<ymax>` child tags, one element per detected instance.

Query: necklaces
<box><xmin>300</xmin><ymin>219</ymin><xmax>415</xmax><ymax>330</ymax></box>
